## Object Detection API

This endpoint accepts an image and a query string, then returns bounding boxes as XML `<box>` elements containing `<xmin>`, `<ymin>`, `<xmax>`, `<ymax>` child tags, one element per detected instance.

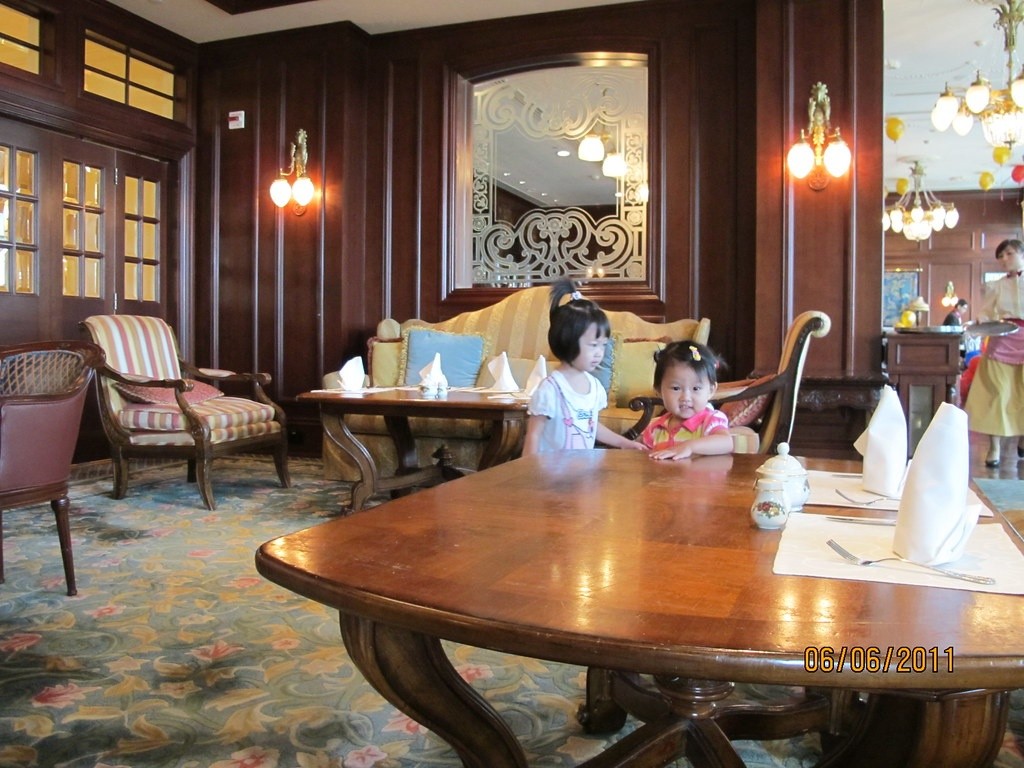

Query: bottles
<box><xmin>755</xmin><ymin>443</ymin><xmax>811</xmax><ymax>512</ymax></box>
<box><xmin>751</xmin><ymin>478</ymin><xmax>788</xmax><ymax>530</ymax></box>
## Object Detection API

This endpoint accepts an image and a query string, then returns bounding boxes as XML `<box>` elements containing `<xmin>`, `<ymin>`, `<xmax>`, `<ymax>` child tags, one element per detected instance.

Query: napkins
<box><xmin>526</xmin><ymin>355</ymin><xmax>547</xmax><ymax>397</ymax></box>
<box><xmin>891</xmin><ymin>402</ymin><xmax>981</xmax><ymax>566</ymax></box>
<box><xmin>487</xmin><ymin>351</ymin><xmax>519</xmax><ymax>391</ymax></box>
<box><xmin>853</xmin><ymin>384</ymin><xmax>913</xmax><ymax>497</ymax></box>
<box><xmin>418</xmin><ymin>352</ymin><xmax>448</xmax><ymax>387</ymax></box>
<box><xmin>337</xmin><ymin>356</ymin><xmax>365</xmax><ymax>391</ymax></box>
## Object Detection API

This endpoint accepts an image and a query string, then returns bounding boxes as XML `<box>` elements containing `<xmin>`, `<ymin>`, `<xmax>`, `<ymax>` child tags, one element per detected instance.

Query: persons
<box><xmin>641</xmin><ymin>339</ymin><xmax>734</xmax><ymax>461</ymax></box>
<box><xmin>521</xmin><ymin>276</ymin><xmax>648</xmax><ymax>456</ymax></box>
<box><xmin>944</xmin><ymin>299</ymin><xmax>968</xmax><ymax>366</ymax></box>
<box><xmin>963</xmin><ymin>239</ymin><xmax>1024</xmax><ymax>466</ymax></box>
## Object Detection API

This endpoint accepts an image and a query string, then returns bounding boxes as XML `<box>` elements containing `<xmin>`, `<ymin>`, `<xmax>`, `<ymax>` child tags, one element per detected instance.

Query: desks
<box><xmin>884</xmin><ymin>329</ymin><xmax>962</xmax><ymax>435</ymax></box>
<box><xmin>293</xmin><ymin>387</ymin><xmax>533</xmax><ymax>511</ymax></box>
<box><xmin>749</xmin><ymin>371</ymin><xmax>888</xmax><ymax>428</ymax></box>
<box><xmin>254</xmin><ymin>448</ymin><xmax>1024</xmax><ymax>768</ymax></box>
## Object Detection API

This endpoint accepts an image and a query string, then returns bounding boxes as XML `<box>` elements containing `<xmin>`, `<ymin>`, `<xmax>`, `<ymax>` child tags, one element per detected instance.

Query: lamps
<box><xmin>787</xmin><ymin>82</ymin><xmax>851</xmax><ymax>192</ymax></box>
<box><xmin>882</xmin><ymin>162</ymin><xmax>961</xmax><ymax>240</ymax></box>
<box><xmin>270</xmin><ymin>129</ymin><xmax>313</xmax><ymax>216</ymax></box>
<box><xmin>576</xmin><ymin>131</ymin><xmax>604</xmax><ymax>162</ymax></box>
<box><xmin>930</xmin><ymin>0</ymin><xmax>1024</xmax><ymax>148</ymax></box>
<box><xmin>602</xmin><ymin>152</ymin><xmax>625</xmax><ymax>178</ymax></box>
<box><xmin>633</xmin><ymin>180</ymin><xmax>650</xmax><ymax>203</ymax></box>
<box><xmin>941</xmin><ymin>281</ymin><xmax>958</xmax><ymax>307</ymax></box>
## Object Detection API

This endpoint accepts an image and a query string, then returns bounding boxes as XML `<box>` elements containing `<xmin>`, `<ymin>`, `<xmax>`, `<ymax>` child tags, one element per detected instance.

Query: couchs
<box><xmin>321</xmin><ymin>285</ymin><xmax>711</xmax><ymax>480</ymax></box>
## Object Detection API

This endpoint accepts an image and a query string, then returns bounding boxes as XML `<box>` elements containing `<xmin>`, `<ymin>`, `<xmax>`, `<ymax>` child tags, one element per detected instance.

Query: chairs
<box><xmin>76</xmin><ymin>312</ymin><xmax>292</xmax><ymax>510</ymax></box>
<box><xmin>0</xmin><ymin>339</ymin><xmax>106</xmax><ymax>594</ymax></box>
<box><xmin>616</xmin><ymin>310</ymin><xmax>833</xmax><ymax>454</ymax></box>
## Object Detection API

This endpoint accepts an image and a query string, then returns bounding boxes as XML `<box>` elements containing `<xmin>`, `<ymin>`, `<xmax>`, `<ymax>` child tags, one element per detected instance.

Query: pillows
<box><xmin>367</xmin><ymin>326</ymin><xmax>673</xmax><ymax>408</ymax></box>
<box><xmin>720</xmin><ymin>373</ymin><xmax>777</xmax><ymax>427</ymax></box>
<box><xmin>113</xmin><ymin>379</ymin><xmax>224</xmax><ymax>405</ymax></box>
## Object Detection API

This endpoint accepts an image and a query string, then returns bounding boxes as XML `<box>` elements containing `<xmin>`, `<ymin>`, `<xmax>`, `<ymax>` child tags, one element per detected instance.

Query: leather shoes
<box><xmin>1018</xmin><ymin>446</ymin><xmax>1024</xmax><ymax>457</ymax></box>
<box><xmin>986</xmin><ymin>447</ymin><xmax>1000</xmax><ymax>467</ymax></box>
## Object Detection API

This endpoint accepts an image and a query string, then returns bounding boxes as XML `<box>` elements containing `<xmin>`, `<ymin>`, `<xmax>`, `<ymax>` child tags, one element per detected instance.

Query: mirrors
<box><xmin>450</xmin><ymin>53</ymin><xmax>649</xmax><ymax>290</ymax></box>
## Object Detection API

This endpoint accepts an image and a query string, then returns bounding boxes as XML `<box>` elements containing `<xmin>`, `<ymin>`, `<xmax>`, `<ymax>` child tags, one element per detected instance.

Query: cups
<box><xmin>419</xmin><ymin>382</ymin><xmax>448</xmax><ymax>397</ymax></box>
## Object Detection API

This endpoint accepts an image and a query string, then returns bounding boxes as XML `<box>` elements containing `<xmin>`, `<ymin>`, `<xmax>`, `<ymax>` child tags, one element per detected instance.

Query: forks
<box><xmin>827</xmin><ymin>540</ymin><xmax>995</xmax><ymax>586</ymax></box>
